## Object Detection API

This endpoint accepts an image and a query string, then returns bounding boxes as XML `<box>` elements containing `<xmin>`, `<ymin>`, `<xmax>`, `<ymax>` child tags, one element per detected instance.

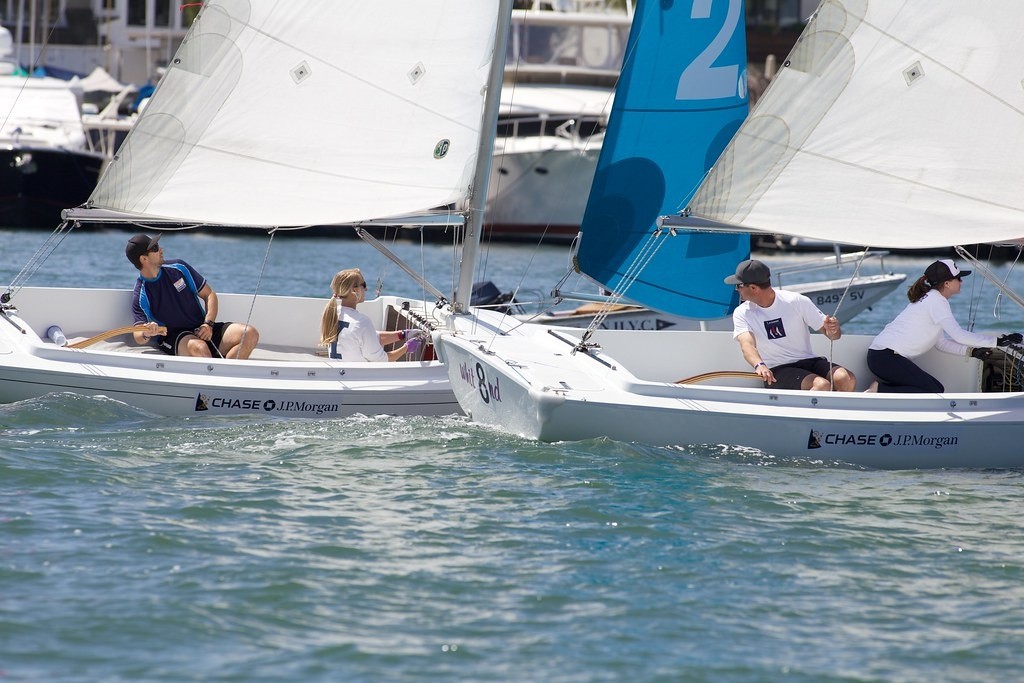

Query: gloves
<box><xmin>997</xmin><ymin>333</ymin><xmax>1023</xmax><ymax>347</ymax></box>
<box><xmin>973</xmin><ymin>348</ymin><xmax>992</xmax><ymax>361</ymax></box>
<box><xmin>405</xmin><ymin>337</ymin><xmax>421</xmax><ymax>353</ymax></box>
<box><xmin>398</xmin><ymin>329</ymin><xmax>422</xmax><ymax>339</ymax></box>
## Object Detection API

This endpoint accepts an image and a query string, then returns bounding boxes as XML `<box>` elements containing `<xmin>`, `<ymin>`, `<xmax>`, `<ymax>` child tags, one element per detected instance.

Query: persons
<box><xmin>125</xmin><ymin>232</ymin><xmax>259</xmax><ymax>360</ymax></box>
<box><xmin>863</xmin><ymin>259</ymin><xmax>1024</xmax><ymax>393</ymax></box>
<box><xmin>724</xmin><ymin>260</ymin><xmax>857</xmax><ymax>392</ymax></box>
<box><xmin>321</xmin><ymin>268</ymin><xmax>420</xmax><ymax>362</ymax></box>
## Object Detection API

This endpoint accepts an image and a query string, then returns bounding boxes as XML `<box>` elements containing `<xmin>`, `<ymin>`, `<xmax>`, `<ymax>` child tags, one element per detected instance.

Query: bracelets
<box><xmin>754</xmin><ymin>362</ymin><xmax>766</xmax><ymax>369</ymax></box>
<box><xmin>397</xmin><ymin>330</ymin><xmax>406</xmax><ymax>340</ymax></box>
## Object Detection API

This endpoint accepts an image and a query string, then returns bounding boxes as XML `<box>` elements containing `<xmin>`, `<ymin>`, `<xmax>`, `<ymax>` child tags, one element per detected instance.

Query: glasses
<box><xmin>735</xmin><ymin>284</ymin><xmax>752</xmax><ymax>290</ymax></box>
<box><xmin>353</xmin><ymin>282</ymin><xmax>366</xmax><ymax>288</ymax></box>
<box><xmin>143</xmin><ymin>244</ymin><xmax>159</xmax><ymax>255</ymax></box>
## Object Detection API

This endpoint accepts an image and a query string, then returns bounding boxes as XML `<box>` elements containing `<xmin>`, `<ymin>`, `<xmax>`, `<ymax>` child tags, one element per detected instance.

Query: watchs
<box><xmin>203</xmin><ymin>319</ymin><xmax>214</xmax><ymax>327</ymax></box>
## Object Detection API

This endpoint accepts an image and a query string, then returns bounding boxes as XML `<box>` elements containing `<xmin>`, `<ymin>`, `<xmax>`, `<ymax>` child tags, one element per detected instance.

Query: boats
<box><xmin>0</xmin><ymin>77</ymin><xmax>109</xmax><ymax>226</ymax></box>
<box><xmin>478</xmin><ymin>84</ymin><xmax>615</xmax><ymax>239</ymax></box>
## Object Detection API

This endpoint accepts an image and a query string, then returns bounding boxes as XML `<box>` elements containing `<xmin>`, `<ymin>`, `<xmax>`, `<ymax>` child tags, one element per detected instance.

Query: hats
<box><xmin>724</xmin><ymin>260</ymin><xmax>771</xmax><ymax>285</ymax></box>
<box><xmin>126</xmin><ymin>233</ymin><xmax>161</xmax><ymax>261</ymax></box>
<box><xmin>925</xmin><ymin>259</ymin><xmax>972</xmax><ymax>285</ymax></box>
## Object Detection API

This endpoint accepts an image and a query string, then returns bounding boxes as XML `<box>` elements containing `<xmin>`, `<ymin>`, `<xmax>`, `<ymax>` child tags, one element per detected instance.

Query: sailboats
<box><xmin>0</xmin><ymin>0</ymin><xmax>903</xmax><ymax>423</ymax></box>
<box><xmin>425</xmin><ymin>0</ymin><xmax>1021</xmax><ymax>474</ymax></box>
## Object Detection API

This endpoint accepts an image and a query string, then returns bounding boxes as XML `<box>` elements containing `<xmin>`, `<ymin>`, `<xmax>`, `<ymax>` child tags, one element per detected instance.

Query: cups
<box><xmin>47</xmin><ymin>324</ymin><xmax>67</xmax><ymax>345</ymax></box>
